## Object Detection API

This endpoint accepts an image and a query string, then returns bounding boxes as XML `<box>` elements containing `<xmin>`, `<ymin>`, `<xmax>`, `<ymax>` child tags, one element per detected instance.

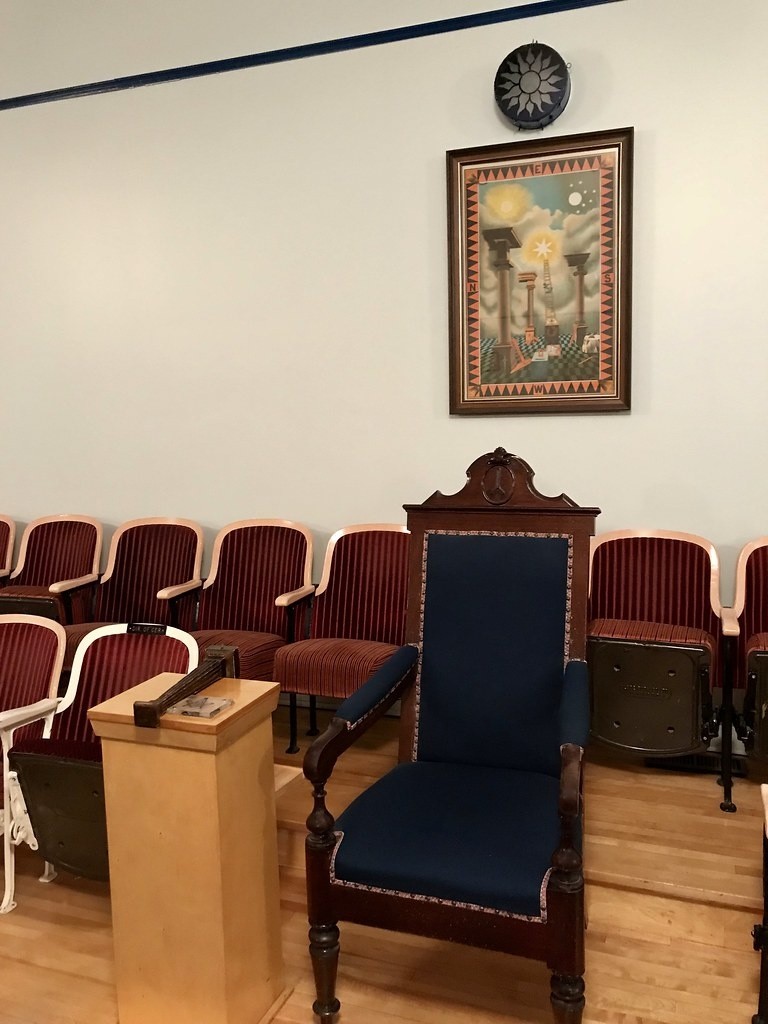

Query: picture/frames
<box><xmin>446</xmin><ymin>126</ymin><xmax>635</xmax><ymax>417</ymax></box>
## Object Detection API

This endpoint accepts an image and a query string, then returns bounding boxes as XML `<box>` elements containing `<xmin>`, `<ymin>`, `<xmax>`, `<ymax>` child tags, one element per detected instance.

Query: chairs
<box><xmin>61</xmin><ymin>516</ymin><xmax>205</xmax><ymax>671</ymax></box>
<box><xmin>587</xmin><ymin>527</ymin><xmax>720</xmax><ymax>760</ymax></box>
<box><xmin>715</xmin><ymin>537</ymin><xmax>768</xmax><ymax>813</ymax></box>
<box><xmin>302</xmin><ymin>446</ymin><xmax>603</xmax><ymax>1024</ymax></box>
<box><xmin>0</xmin><ymin>514</ymin><xmax>17</xmax><ymax>588</ymax></box>
<box><xmin>184</xmin><ymin>518</ymin><xmax>314</xmax><ymax>681</ymax></box>
<box><xmin>270</xmin><ymin>523</ymin><xmax>411</xmax><ymax>755</ymax></box>
<box><xmin>0</xmin><ymin>613</ymin><xmax>66</xmax><ymax>915</ymax></box>
<box><xmin>7</xmin><ymin>622</ymin><xmax>200</xmax><ymax>883</ymax></box>
<box><xmin>0</xmin><ymin>514</ymin><xmax>105</xmax><ymax>625</ymax></box>
